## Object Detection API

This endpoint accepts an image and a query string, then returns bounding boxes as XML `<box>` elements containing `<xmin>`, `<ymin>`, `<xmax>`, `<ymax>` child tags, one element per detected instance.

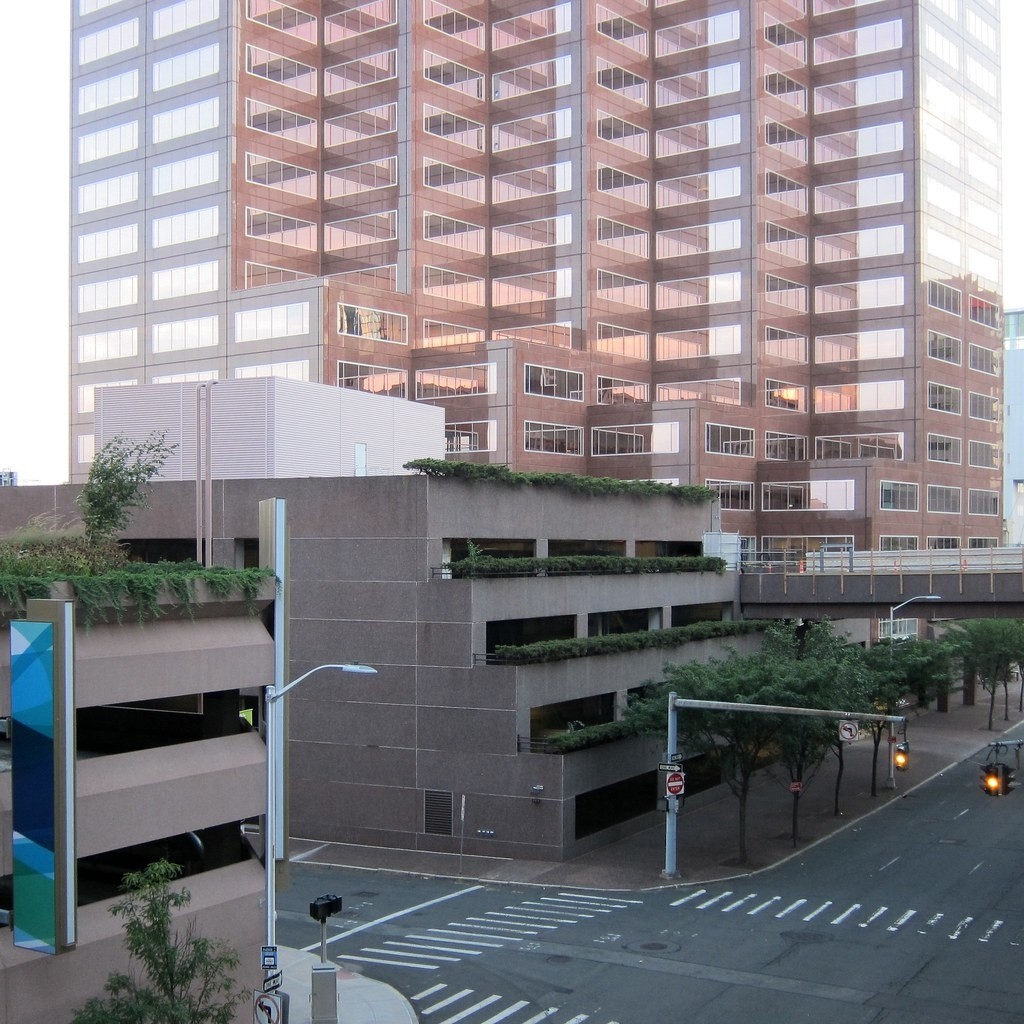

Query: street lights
<box><xmin>265</xmin><ymin>664</ymin><xmax>378</xmax><ymax>995</ymax></box>
<box><xmin>890</xmin><ymin>596</ymin><xmax>941</xmax><ymax>661</ymax></box>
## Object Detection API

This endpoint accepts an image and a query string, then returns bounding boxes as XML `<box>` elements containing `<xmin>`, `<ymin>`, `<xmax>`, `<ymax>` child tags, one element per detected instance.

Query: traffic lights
<box><xmin>997</xmin><ymin>763</ymin><xmax>1015</xmax><ymax>795</ymax></box>
<box><xmin>981</xmin><ymin>763</ymin><xmax>997</xmax><ymax>796</ymax></box>
<box><xmin>895</xmin><ymin>743</ymin><xmax>910</xmax><ymax>772</ymax></box>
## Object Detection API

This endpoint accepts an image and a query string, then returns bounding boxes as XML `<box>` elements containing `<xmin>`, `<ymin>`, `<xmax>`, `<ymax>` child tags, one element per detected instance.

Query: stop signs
<box><xmin>667</xmin><ymin>772</ymin><xmax>683</xmax><ymax>795</ymax></box>
